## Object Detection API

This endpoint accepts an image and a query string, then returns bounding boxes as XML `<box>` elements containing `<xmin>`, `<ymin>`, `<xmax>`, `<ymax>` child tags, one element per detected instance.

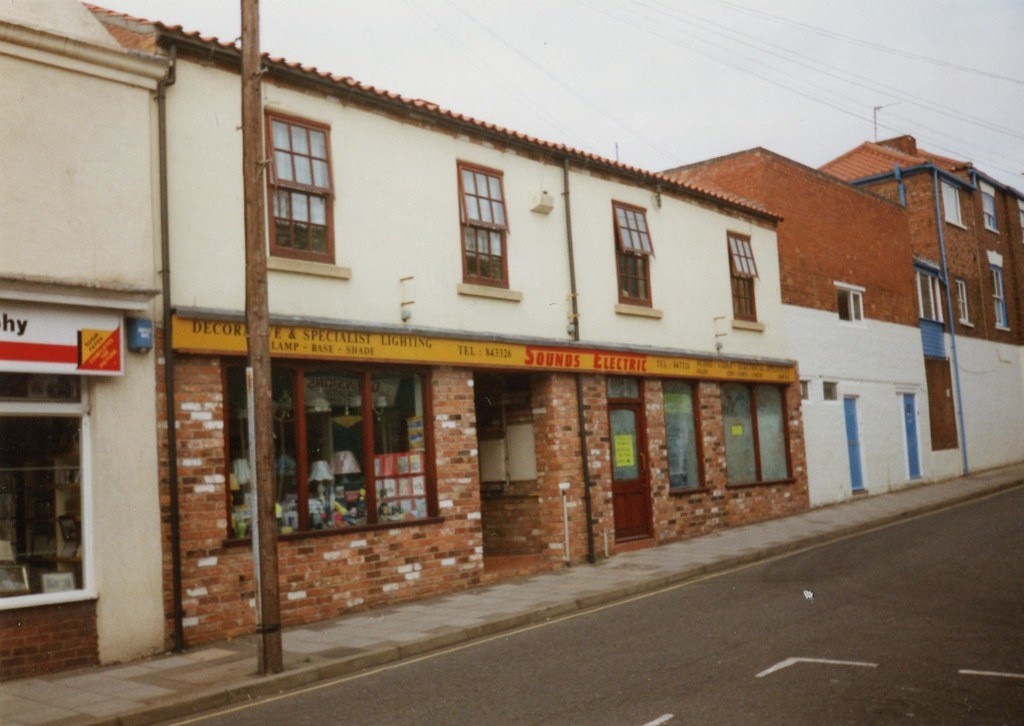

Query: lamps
<box><xmin>308</xmin><ymin>461</ymin><xmax>334</xmax><ymax>507</ymax></box>
<box><xmin>329</xmin><ymin>450</ymin><xmax>362</xmax><ymax>485</ymax></box>
